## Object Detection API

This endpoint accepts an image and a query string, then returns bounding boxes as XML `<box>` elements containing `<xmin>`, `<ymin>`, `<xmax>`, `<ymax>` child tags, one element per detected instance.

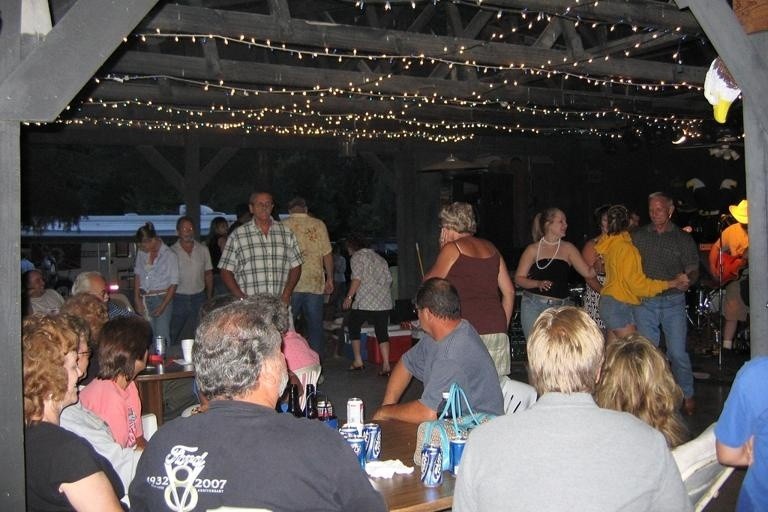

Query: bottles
<box><xmin>288</xmin><ymin>384</ymin><xmax>316</xmax><ymax>419</ymax></box>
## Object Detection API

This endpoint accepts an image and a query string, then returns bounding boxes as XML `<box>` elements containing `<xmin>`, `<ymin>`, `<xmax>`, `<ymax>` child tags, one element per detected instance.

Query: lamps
<box><xmin>414</xmin><ymin>142</ymin><xmax>490</xmax><ymax>176</ymax></box>
<box><xmin>707</xmin><ymin>145</ymin><xmax>740</xmax><ymax>162</ymax></box>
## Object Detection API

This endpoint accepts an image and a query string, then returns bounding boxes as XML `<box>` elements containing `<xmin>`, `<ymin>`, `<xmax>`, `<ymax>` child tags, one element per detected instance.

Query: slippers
<box><xmin>346</xmin><ymin>364</ymin><xmax>365</xmax><ymax>370</ymax></box>
<box><xmin>378</xmin><ymin>367</ymin><xmax>391</xmax><ymax>376</ymax></box>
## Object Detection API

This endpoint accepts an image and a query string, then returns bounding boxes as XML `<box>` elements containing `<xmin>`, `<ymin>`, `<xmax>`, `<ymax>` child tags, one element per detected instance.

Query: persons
<box><xmin>205</xmin><ymin>189</ymin><xmax>334</xmax><ymax>384</ymax></box>
<box><xmin>24</xmin><ymin>270</ymin><xmax>133</xmax><ymax>387</ymax></box>
<box><xmin>134</xmin><ymin>216</ymin><xmax>213</xmax><ymax>349</ymax></box>
<box><xmin>714</xmin><ymin>353</ymin><xmax>767</xmax><ymax>510</ymax></box>
<box><xmin>419</xmin><ymin>201</ymin><xmax>515</xmax><ymax>376</ymax></box>
<box><xmin>373</xmin><ymin>278</ymin><xmax>505</xmax><ymax>424</ymax></box>
<box><xmin>42</xmin><ymin>304</ymin><xmax>141</xmax><ymax>509</ymax></box>
<box><xmin>126</xmin><ymin>298</ymin><xmax>388</xmax><ymax>510</ymax></box>
<box><xmin>21</xmin><ymin>313</ymin><xmax>131</xmax><ymax>511</ymax></box>
<box><xmin>342</xmin><ymin>238</ymin><xmax>393</xmax><ymax>375</ymax></box>
<box><xmin>451</xmin><ymin>307</ymin><xmax>694</xmax><ymax>511</ymax></box>
<box><xmin>514</xmin><ymin>194</ymin><xmax>748</xmax><ymax>414</ymax></box>
<box><xmin>595</xmin><ymin>333</ymin><xmax>693</xmax><ymax>448</ymax></box>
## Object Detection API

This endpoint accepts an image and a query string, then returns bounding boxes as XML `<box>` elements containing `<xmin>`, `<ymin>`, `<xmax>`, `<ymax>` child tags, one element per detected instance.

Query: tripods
<box><xmin>685</xmin><ymin>281</ymin><xmax>719</xmax><ymax>334</ymax></box>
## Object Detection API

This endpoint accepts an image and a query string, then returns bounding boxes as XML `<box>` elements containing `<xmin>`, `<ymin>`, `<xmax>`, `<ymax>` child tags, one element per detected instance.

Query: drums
<box><xmin>686</xmin><ymin>285</ymin><xmax>714</xmax><ymax>309</ymax></box>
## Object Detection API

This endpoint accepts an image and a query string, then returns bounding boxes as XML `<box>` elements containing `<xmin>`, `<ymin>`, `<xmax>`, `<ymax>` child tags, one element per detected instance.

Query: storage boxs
<box><xmin>342</xmin><ymin>324</ymin><xmax>413</xmax><ymax>365</ymax></box>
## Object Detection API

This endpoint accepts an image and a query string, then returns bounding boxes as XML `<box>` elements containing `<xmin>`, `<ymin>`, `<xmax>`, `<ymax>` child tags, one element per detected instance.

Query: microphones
<box><xmin>720</xmin><ymin>213</ymin><xmax>727</xmax><ymax>223</ymax></box>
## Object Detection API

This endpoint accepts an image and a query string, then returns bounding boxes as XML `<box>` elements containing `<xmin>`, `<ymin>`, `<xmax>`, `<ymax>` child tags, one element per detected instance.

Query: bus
<box><xmin>21</xmin><ymin>204</ymin><xmax>314</xmax><ymax>304</ymax></box>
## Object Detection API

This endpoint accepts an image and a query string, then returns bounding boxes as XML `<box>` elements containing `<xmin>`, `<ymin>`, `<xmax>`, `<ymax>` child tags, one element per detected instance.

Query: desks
<box><xmin>306</xmin><ymin>418</ymin><xmax>464</xmax><ymax>512</ymax></box>
<box><xmin>118</xmin><ymin>355</ymin><xmax>195</xmax><ymax>429</ymax></box>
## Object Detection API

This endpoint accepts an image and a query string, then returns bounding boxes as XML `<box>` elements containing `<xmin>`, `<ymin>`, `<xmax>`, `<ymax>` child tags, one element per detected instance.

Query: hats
<box><xmin>729</xmin><ymin>200</ymin><xmax>748</xmax><ymax>224</ymax></box>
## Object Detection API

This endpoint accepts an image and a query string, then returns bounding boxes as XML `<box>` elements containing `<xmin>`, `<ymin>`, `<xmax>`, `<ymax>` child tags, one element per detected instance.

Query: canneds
<box><xmin>317</xmin><ymin>399</ymin><xmax>332</xmax><ymax>417</ymax></box>
<box><xmin>347</xmin><ymin>437</ymin><xmax>365</xmax><ymax>471</ymax></box>
<box><xmin>449</xmin><ymin>439</ymin><xmax>466</xmax><ymax>475</ymax></box>
<box><xmin>339</xmin><ymin>427</ymin><xmax>357</xmax><ymax>439</ymax></box>
<box><xmin>362</xmin><ymin>424</ymin><xmax>381</xmax><ymax>460</ymax></box>
<box><xmin>421</xmin><ymin>444</ymin><xmax>443</xmax><ymax>488</ymax></box>
<box><xmin>151</xmin><ymin>336</ymin><xmax>167</xmax><ymax>360</ymax></box>
<box><xmin>347</xmin><ymin>397</ymin><xmax>363</xmax><ymax>427</ymax></box>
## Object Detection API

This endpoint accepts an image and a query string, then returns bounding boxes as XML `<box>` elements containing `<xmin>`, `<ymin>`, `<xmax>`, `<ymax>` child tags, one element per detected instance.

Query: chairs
<box><xmin>497</xmin><ymin>380</ymin><xmax>537</xmax><ymax>415</ymax></box>
<box><xmin>674</xmin><ymin>421</ymin><xmax>732</xmax><ymax>512</ymax></box>
<box><xmin>109</xmin><ymin>292</ymin><xmax>136</xmax><ymax>314</ymax></box>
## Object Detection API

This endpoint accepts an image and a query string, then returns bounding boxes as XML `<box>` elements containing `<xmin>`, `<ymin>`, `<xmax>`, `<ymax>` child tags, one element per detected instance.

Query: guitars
<box><xmin>712</xmin><ymin>247</ymin><xmax>747</xmax><ymax>286</ymax></box>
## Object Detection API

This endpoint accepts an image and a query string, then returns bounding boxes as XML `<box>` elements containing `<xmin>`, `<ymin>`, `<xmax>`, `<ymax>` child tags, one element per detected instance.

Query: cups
<box><xmin>180</xmin><ymin>339</ymin><xmax>195</xmax><ymax>362</ymax></box>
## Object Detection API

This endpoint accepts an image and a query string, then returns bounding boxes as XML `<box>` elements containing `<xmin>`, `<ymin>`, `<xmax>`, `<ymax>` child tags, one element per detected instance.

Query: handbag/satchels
<box><xmin>414</xmin><ymin>412</ymin><xmax>499</xmax><ymax>471</ymax></box>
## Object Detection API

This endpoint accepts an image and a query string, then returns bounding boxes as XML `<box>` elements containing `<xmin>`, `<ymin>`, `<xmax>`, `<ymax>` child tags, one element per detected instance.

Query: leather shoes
<box><xmin>684</xmin><ymin>397</ymin><xmax>696</xmax><ymax>415</ymax></box>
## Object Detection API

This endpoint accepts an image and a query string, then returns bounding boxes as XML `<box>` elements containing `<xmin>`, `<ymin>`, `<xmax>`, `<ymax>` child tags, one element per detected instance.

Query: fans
<box><xmin>670</xmin><ymin>127</ymin><xmax>744</xmax><ymax>151</ymax></box>
